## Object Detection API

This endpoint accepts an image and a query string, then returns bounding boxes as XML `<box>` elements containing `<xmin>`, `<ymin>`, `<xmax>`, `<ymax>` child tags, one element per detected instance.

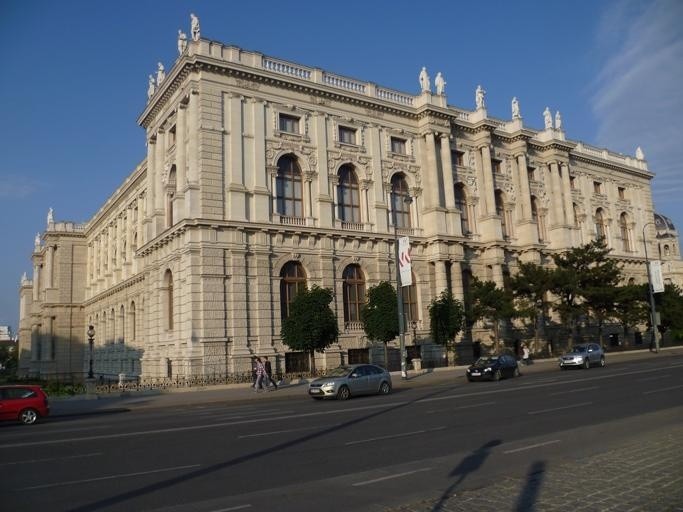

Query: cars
<box><xmin>307</xmin><ymin>363</ymin><xmax>393</xmax><ymax>401</ymax></box>
<box><xmin>559</xmin><ymin>343</ymin><xmax>606</xmax><ymax>371</ymax></box>
<box><xmin>0</xmin><ymin>384</ymin><xmax>50</xmax><ymax>425</ymax></box>
<box><xmin>466</xmin><ymin>353</ymin><xmax>520</xmax><ymax>382</ymax></box>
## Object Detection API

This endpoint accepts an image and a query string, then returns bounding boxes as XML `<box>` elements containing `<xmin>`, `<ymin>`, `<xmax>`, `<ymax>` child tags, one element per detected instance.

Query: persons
<box><xmin>521</xmin><ymin>344</ymin><xmax>530</xmax><ymax>366</ymax></box>
<box><xmin>250</xmin><ymin>354</ymin><xmax>277</xmax><ymax>393</ymax></box>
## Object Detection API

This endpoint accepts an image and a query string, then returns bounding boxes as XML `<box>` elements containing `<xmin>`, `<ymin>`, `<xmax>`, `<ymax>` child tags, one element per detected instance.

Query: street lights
<box><xmin>390</xmin><ymin>186</ymin><xmax>413</xmax><ymax>380</ymax></box>
<box><xmin>85</xmin><ymin>325</ymin><xmax>98</xmax><ymax>401</ymax></box>
<box><xmin>643</xmin><ymin>221</ymin><xmax>664</xmax><ymax>353</ymax></box>
